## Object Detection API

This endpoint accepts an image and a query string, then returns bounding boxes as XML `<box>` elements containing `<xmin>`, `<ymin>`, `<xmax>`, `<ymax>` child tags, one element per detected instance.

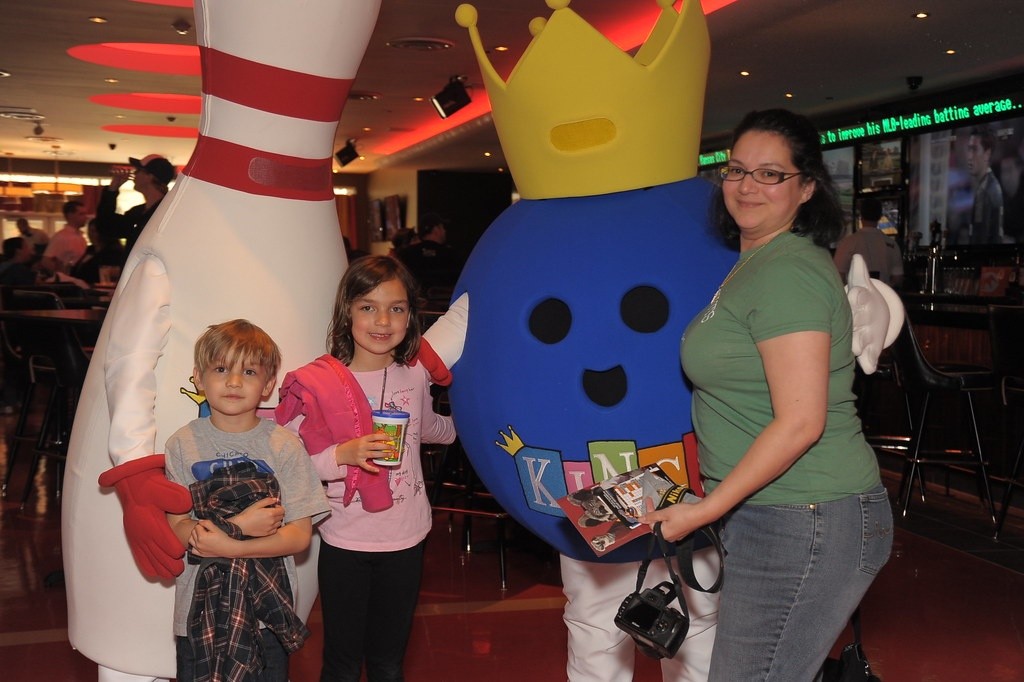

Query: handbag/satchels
<box><xmin>821</xmin><ymin>642</ymin><xmax>881</xmax><ymax>682</ymax></box>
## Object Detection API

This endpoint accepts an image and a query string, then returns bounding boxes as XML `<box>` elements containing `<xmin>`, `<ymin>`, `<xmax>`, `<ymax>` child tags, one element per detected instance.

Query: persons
<box><xmin>0</xmin><ymin>154</ymin><xmax>176</xmax><ymax>418</ymax></box>
<box><xmin>964</xmin><ymin>126</ymin><xmax>1024</xmax><ymax>246</ymax></box>
<box><xmin>834</xmin><ymin>198</ymin><xmax>904</xmax><ymax>297</ymax></box>
<box><xmin>636</xmin><ymin>110</ymin><xmax>894</xmax><ymax>682</ymax></box>
<box><xmin>335</xmin><ymin>217</ymin><xmax>463</xmax><ymax>313</ymax></box>
<box><xmin>279</xmin><ymin>255</ymin><xmax>456</xmax><ymax>682</ymax></box>
<box><xmin>165</xmin><ymin>312</ymin><xmax>333</xmax><ymax>682</ymax></box>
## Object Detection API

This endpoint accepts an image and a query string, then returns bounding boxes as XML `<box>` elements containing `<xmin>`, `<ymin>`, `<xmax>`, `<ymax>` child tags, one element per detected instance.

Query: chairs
<box><xmin>889</xmin><ymin>305</ymin><xmax>1024</xmax><ymax>548</ymax></box>
<box><xmin>0</xmin><ymin>286</ymin><xmax>92</xmax><ymax>512</ymax></box>
<box><xmin>419</xmin><ymin>442</ymin><xmax>510</xmax><ymax>590</ymax></box>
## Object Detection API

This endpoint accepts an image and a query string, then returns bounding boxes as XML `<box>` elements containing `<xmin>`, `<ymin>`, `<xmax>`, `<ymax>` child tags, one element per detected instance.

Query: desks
<box><xmin>0</xmin><ymin>306</ymin><xmax>107</xmax><ymax>325</ymax></box>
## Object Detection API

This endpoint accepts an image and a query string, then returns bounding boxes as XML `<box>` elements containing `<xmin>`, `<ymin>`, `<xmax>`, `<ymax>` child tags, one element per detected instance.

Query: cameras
<box><xmin>614</xmin><ymin>580</ymin><xmax>689</xmax><ymax>660</ymax></box>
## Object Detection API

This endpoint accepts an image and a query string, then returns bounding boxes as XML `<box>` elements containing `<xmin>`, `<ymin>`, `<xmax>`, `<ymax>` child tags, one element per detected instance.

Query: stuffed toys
<box><xmin>59</xmin><ymin>0</ymin><xmax>471</xmax><ymax>682</ymax></box>
<box><xmin>422</xmin><ymin>0</ymin><xmax>907</xmax><ymax>682</ymax></box>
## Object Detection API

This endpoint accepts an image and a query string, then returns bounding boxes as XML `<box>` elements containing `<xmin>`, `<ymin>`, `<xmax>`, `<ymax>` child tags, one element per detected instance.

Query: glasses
<box><xmin>718</xmin><ymin>165</ymin><xmax>803</xmax><ymax>185</ymax></box>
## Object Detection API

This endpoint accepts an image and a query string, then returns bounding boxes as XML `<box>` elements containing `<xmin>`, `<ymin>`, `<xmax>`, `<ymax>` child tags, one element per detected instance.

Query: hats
<box><xmin>129</xmin><ymin>154</ymin><xmax>174</xmax><ymax>183</ymax></box>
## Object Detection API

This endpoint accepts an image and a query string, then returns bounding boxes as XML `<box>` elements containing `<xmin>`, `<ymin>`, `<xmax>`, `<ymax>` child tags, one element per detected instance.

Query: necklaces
<box><xmin>710</xmin><ymin>235</ymin><xmax>774</xmax><ymax>304</ymax></box>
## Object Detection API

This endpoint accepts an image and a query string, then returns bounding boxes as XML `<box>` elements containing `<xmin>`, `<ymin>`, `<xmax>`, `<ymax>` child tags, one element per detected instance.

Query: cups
<box><xmin>371</xmin><ymin>410</ymin><xmax>410</xmax><ymax>466</ymax></box>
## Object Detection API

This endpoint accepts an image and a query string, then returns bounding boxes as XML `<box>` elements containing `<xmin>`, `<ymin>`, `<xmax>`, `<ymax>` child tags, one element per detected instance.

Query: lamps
<box><xmin>335</xmin><ymin>136</ymin><xmax>361</xmax><ymax>166</ymax></box>
<box><xmin>430</xmin><ymin>77</ymin><xmax>472</xmax><ymax>121</ymax></box>
